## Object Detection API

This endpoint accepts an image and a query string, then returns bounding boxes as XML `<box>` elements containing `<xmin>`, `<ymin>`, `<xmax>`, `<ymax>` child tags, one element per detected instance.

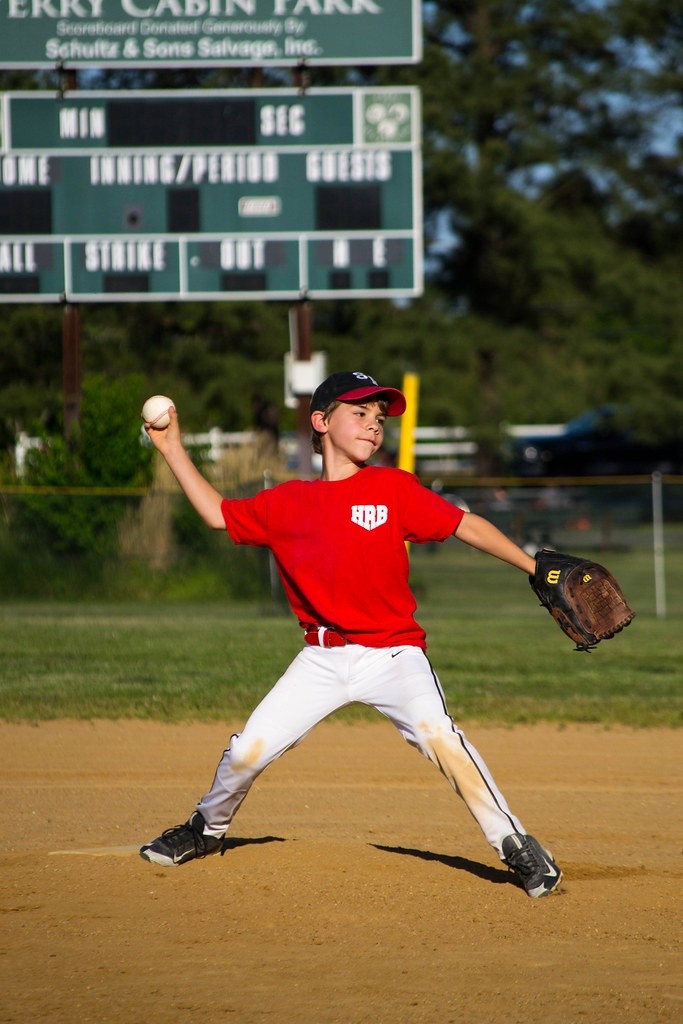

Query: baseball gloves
<box><xmin>529</xmin><ymin>547</ymin><xmax>638</xmax><ymax>650</ymax></box>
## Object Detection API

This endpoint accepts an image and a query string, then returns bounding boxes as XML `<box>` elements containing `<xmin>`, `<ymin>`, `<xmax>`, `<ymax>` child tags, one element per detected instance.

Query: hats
<box><xmin>310</xmin><ymin>371</ymin><xmax>406</xmax><ymax>417</ymax></box>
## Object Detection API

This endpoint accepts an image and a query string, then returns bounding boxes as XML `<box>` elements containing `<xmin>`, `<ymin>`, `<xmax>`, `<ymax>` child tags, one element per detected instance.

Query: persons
<box><xmin>138</xmin><ymin>371</ymin><xmax>634</xmax><ymax>899</ymax></box>
<box><xmin>250</xmin><ymin>392</ymin><xmax>280</xmax><ymax>432</ymax></box>
<box><xmin>522</xmin><ymin>448</ymin><xmax>558</xmax><ymax>489</ymax></box>
<box><xmin>469</xmin><ymin>440</ymin><xmax>505</xmax><ymax>515</ymax></box>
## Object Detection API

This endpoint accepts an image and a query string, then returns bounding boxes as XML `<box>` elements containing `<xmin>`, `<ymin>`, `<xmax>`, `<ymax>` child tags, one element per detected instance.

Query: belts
<box><xmin>304</xmin><ymin>625</ymin><xmax>352</xmax><ymax>647</ymax></box>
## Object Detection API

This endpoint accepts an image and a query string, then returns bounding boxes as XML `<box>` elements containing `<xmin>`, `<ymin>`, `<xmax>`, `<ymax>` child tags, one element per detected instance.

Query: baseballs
<box><xmin>142</xmin><ymin>395</ymin><xmax>176</xmax><ymax>429</ymax></box>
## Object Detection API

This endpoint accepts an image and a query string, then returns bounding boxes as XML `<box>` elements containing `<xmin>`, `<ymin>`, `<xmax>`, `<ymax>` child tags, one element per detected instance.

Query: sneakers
<box><xmin>138</xmin><ymin>811</ymin><xmax>226</xmax><ymax>867</ymax></box>
<box><xmin>500</xmin><ymin>833</ymin><xmax>563</xmax><ymax>900</ymax></box>
